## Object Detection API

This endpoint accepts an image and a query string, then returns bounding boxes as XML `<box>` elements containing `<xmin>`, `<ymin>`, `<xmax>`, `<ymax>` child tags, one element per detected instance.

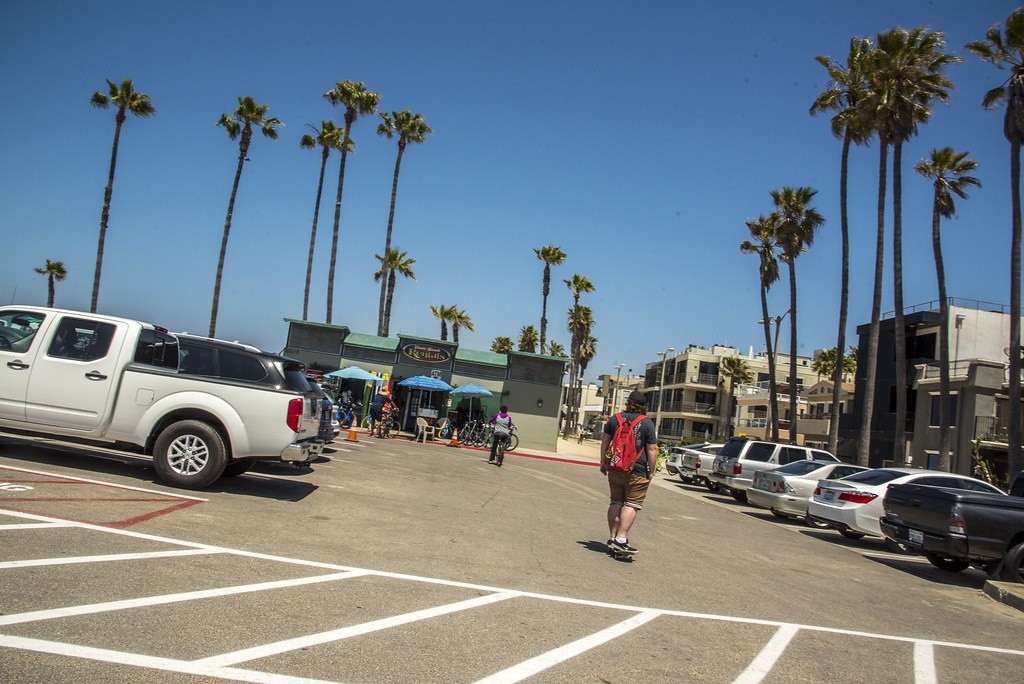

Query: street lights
<box><xmin>758</xmin><ymin>308</ymin><xmax>799</xmax><ymax>442</ymax></box>
<box><xmin>655</xmin><ymin>348</ymin><xmax>674</xmax><ymax>438</ymax></box>
<box><xmin>614</xmin><ymin>364</ymin><xmax>625</xmax><ymax>414</ymax></box>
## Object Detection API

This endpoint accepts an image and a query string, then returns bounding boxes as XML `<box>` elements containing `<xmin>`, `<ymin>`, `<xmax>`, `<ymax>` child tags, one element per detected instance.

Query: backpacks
<box><xmin>603</xmin><ymin>412</ymin><xmax>651</xmax><ymax>475</ymax></box>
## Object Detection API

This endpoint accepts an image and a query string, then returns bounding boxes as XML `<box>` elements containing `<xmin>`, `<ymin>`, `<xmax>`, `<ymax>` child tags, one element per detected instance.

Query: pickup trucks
<box><xmin>879</xmin><ymin>469</ymin><xmax>1024</xmax><ymax>583</ymax></box>
<box><xmin>0</xmin><ymin>305</ymin><xmax>310</xmax><ymax>490</ymax></box>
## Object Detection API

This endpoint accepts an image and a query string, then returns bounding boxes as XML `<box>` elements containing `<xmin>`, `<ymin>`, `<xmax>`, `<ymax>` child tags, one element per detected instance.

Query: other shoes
<box><xmin>488</xmin><ymin>461</ymin><xmax>493</xmax><ymax>464</ymax></box>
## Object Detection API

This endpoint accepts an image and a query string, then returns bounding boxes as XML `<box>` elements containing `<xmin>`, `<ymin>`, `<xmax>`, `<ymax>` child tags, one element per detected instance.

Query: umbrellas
<box><xmin>449</xmin><ymin>383</ymin><xmax>493</xmax><ymax>420</ymax></box>
<box><xmin>323</xmin><ymin>365</ymin><xmax>382</xmax><ymax>380</ymax></box>
<box><xmin>396</xmin><ymin>376</ymin><xmax>454</xmax><ymax>431</ymax></box>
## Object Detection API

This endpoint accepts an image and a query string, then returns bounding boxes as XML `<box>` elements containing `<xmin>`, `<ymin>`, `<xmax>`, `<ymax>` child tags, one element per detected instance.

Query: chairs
<box><xmin>416</xmin><ymin>418</ymin><xmax>435</xmax><ymax>444</ymax></box>
<box><xmin>82</xmin><ymin>332</ymin><xmax>105</xmax><ymax>359</ymax></box>
<box><xmin>59</xmin><ymin>327</ymin><xmax>79</xmax><ymax>358</ymax></box>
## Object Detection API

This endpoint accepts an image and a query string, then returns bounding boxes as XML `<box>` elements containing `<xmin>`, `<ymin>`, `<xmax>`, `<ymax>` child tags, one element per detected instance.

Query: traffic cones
<box><xmin>343</xmin><ymin>417</ymin><xmax>359</xmax><ymax>442</ymax></box>
<box><xmin>448</xmin><ymin>427</ymin><xmax>458</xmax><ymax>447</ymax></box>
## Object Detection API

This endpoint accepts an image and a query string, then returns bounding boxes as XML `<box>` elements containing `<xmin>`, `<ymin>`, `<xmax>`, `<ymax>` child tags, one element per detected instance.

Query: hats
<box><xmin>628</xmin><ymin>391</ymin><xmax>647</xmax><ymax>408</ymax></box>
<box><xmin>378</xmin><ymin>386</ymin><xmax>390</xmax><ymax>395</ymax></box>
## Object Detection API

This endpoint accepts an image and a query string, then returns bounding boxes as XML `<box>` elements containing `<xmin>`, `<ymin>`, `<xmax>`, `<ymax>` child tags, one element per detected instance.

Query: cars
<box><xmin>746</xmin><ymin>459</ymin><xmax>873</xmax><ymax>529</ymax></box>
<box><xmin>303</xmin><ymin>375</ymin><xmax>334</xmax><ymax>442</ymax></box>
<box><xmin>317</xmin><ymin>384</ymin><xmax>345</xmax><ymax>437</ymax></box>
<box><xmin>679</xmin><ymin>443</ymin><xmax>731</xmax><ymax>496</ymax></box>
<box><xmin>665</xmin><ymin>443</ymin><xmax>709</xmax><ymax>486</ymax></box>
<box><xmin>808</xmin><ymin>468</ymin><xmax>1009</xmax><ymax>555</ymax></box>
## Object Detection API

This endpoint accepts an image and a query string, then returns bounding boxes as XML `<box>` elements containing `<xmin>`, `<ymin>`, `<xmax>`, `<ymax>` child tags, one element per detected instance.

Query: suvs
<box><xmin>707</xmin><ymin>435</ymin><xmax>841</xmax><ymax>502</ymax></box>
<box><xmin>83</xmin><ymin>331</ymin><xmax>312</xmax><ymax>476</ymax></box>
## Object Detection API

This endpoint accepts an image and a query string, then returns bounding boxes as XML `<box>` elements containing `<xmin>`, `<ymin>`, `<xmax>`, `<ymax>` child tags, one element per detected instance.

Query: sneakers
<box><xmin>611</xmin><ymin>539</ymin><xmax>640</xmax><ymax>553</ymax></box>
<box><xmin>607</xmin><ymin>539</ymin><xmax>615</xmax><ymax>549</ymax></box>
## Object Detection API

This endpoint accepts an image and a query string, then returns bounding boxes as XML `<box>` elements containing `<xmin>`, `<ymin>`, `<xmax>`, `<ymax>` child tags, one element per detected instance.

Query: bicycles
<box><xmin>378</xmin><ymin>407</ymin><xmax>401</xmax><ymax>439</ymax></box>
<box><xmin>338</xmin><ymin>395</ymin><xmax>363</xmax><ymax>429</ymax></box>
<box><xmin>457</xmin><ymin>419</ymin><xmax>519</xmax><ymax>467</ymax></box>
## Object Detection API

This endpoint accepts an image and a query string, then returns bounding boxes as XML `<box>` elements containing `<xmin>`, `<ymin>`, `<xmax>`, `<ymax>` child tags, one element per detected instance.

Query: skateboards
<box><xmin>606</xmin><ymin>543</ymin><xmax>640</xmax><ymax>563</ymax></box>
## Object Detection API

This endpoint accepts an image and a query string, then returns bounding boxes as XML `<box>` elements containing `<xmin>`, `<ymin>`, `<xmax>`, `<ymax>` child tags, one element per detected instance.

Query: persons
<box><xmin>600</xmin><ymin>391</ymin><xmax>657</xmax><ymax>553</ymax></box>
<box><xmin>577</xmin><ymin>432</ymin><xmax>584</xmax><ymax>444</ymax></box>
<box><xmin>488</xmin><ymin>405</ymin><xmax>511</xmax><ymax>463</ymax></box>
<box><xmin>370</xmin><ymin>387</ymin><xmax>399</xmax><ymax>439</ymax></box>
